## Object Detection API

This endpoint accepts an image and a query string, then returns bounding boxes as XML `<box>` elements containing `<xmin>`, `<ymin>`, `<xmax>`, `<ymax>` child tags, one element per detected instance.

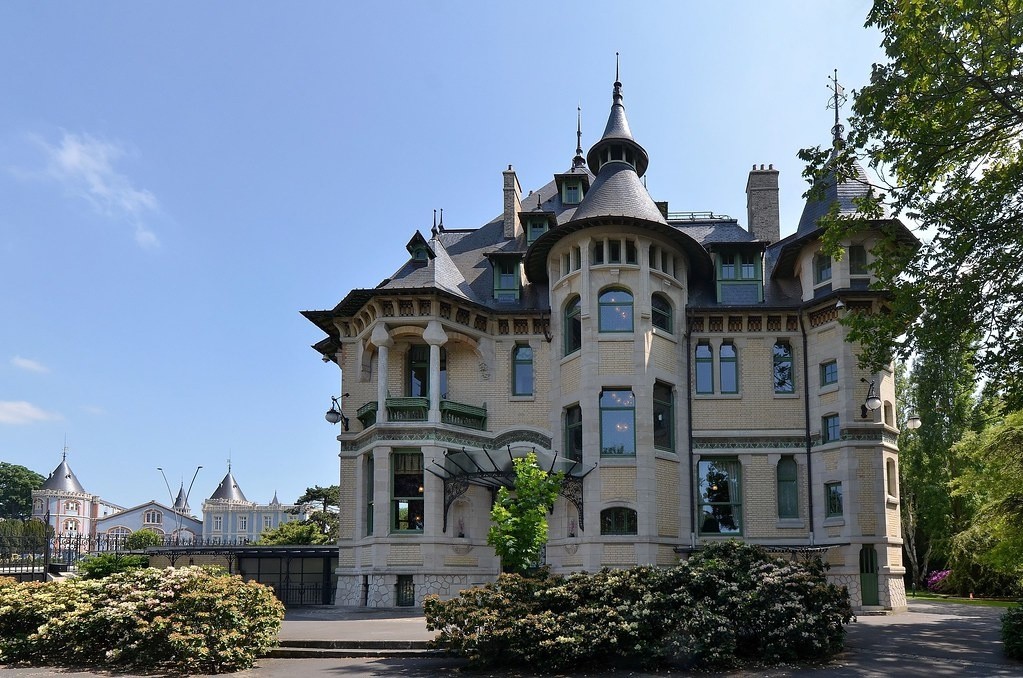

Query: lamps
<box><xmin>322</xmin><ymin>353</ymin><xmax>330</xmax><ymax>363</ymax></box>
<box><xmin>860</xmin><ymin>378</ymin><xmax>881</xmax><ymax>419</ymax></box>
<box><xmin>325</xmin><ymin>393</ymin><xmax>349</xmax><ymax>432</ymax></box>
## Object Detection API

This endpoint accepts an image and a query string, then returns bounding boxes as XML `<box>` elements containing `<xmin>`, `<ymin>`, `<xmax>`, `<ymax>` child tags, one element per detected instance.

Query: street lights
<box><xmin>180</xmin><ymin>466</ymin><xmax>203</xmax><ymax>534</ymax></box>
<box><xmin>156</xmin><ymin>467</ymin><xmax>178</xmax><ymax>542</ymax></box>
